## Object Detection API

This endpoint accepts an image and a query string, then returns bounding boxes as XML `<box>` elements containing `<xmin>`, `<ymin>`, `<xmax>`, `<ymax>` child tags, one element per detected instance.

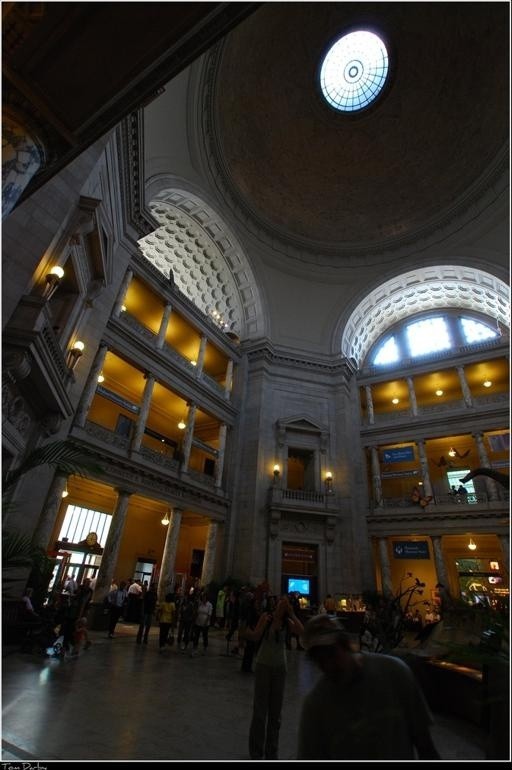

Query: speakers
<box><xmin>203</xmin><ymin>457</ymin><xmax>215</xmax><ymax>477</ymax></box>
<box><xmin>189</xmin><ymin>548</ymin><xmax>205</xmax><ymax>579</ymax></box>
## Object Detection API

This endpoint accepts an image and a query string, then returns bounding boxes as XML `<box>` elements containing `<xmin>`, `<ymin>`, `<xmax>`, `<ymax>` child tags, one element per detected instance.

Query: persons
<box><xmin>411</xmin><ymin>485</ymin><xmax>422</xmax><ymax>505</ymax></box>
<box><xmin>322</xmin><ymin>593</ymin><xmax>337</xmax><ymax>615</ymax></box>
<box><xmin>293</xmin><ymin>612</ymin><xmax>443</xmax><ymax>760</ymax></box>
<box><xmin>448</xmin><ymin>484</ymin><xmax>459</xmax><ymax>505</ymax></box>
<box><xmin>489</xmin><ymin>592</ymin><xmax>499</xmax><ymax>611</ymax></box>
<box><xmin>404</xmin><ymin>605</ymin><xmax>441</xmax><ymax>627</ymax></box>
<box><xmin>246</xmin><ymin>594</ymin><xmax>306</xmax><ymax>759</ymax></box>
<box><xmin>21</xmin><ymin>574</ymin><xmax>305</xmax><ymax>674</ymax></box>
<box><xmin>457</xmin><ymin>485</ymin><xmax>469</xmax><ymax>505</ymax></box>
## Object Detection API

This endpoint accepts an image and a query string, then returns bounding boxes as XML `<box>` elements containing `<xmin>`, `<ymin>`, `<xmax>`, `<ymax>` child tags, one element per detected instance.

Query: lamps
<box><xmin>69</xmin><ymin>338</ymin><xmax>86</xmax><ymax>370</ymax></box>
<box><xmin>177</xmin><ymin>416</ymin><xmax>187</xmax><ymax>429</ymax></box>
<box><xmin>61</xmin><ymin>481</ymin><xmax>70</xmax><ymax>502</ymax></box>
<box><xmin>272</xmin><ymin>462</ymin><xmax>281</xmax><ymax>482</ymax></box>
<box><xmin>466</xmin><ymin>537</ymin><xmax>479</xmax><ymax>551</ymax></box>
<box><xmin>45</xmin><ymin>263</ymin><xmax>66</xmax><ymax>299</ymax></box>
<box><xmin>325</xmin><ymin>471</ymin><xmax>334</xmax><ymax>489</ymax></box>
<box><xmin>161</xmin><ymin>511</ymin><xmax>171</xmax><ymax>526</ymax></box>
<box><xmin>447</xmin><ymin>446</ymin><xmax>457</xmax><ymax>459</ymax></box>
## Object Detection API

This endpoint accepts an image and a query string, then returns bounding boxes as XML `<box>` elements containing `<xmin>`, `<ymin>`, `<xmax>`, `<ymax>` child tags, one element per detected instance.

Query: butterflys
<box><xmin>410</xmin><ymin>487</ymin><xmax>433</xmax><ymax>508</ymax></box>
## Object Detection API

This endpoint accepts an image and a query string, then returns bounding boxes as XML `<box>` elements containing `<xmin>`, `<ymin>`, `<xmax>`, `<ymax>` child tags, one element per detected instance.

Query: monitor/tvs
<box><xmin>287</xmin><ymin>578</ymin><xmax>312</xmax><ymax>595</ymax></box>
